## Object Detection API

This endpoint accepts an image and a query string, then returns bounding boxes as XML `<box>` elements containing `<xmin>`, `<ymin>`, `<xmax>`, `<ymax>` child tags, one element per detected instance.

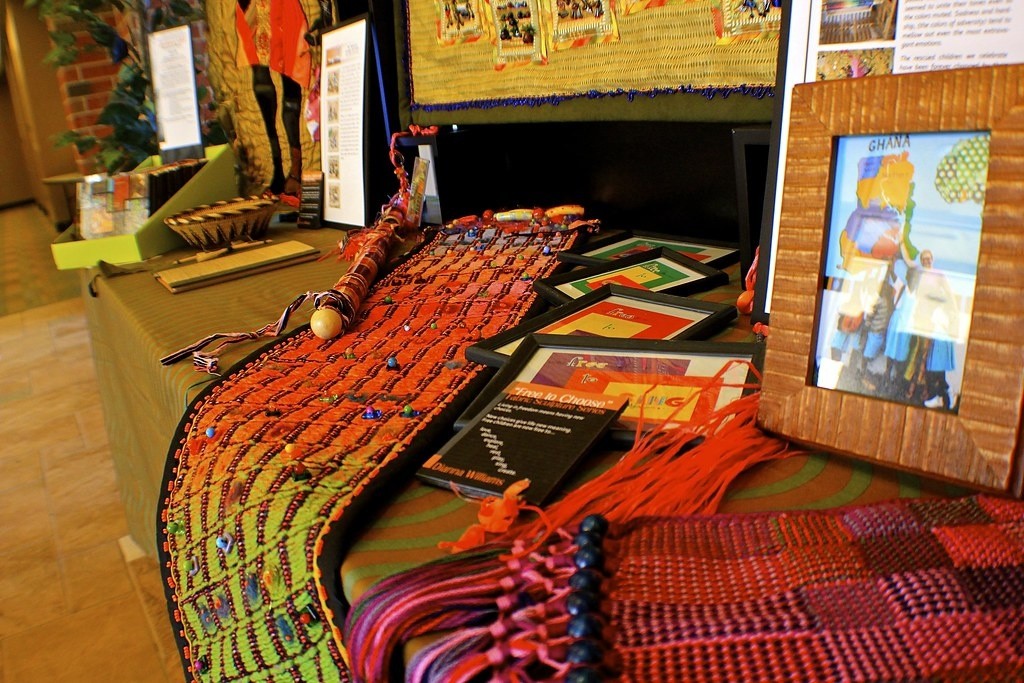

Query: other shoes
<box><xmin>947</xmin><ymin>387</ymin><xmax>957</xmax><ymax>410</ymax></box>
<box><xmin>924</xmin><ymin>395</ymin><xmax>944</xmax><ymax>408</ymax></box>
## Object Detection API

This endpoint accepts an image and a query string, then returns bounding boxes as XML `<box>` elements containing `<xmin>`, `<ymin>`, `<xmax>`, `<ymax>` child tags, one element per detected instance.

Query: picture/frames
<box><xmin>453</xmin><ymin>229</ymin><xmax>767</xmax><ymax>452</ymax></box>
<box><xmin>755</xmin><ymin>65</ymin><xmax>1024</xmax><ymax>499</ymax></box>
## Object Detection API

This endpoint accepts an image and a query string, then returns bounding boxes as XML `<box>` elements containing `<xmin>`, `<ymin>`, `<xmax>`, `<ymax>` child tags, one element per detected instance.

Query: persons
<box><xmin>830</xmin><ymin>202</ymin><xmax>957</xmax><ymax>408</ymax></box>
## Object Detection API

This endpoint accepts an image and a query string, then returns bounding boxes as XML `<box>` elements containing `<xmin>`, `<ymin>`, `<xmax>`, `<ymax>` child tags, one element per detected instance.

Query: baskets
<box><xmin>164</xmin><ymin>193</ymin><xmax>281</xmax><ymax>247</ymax></box>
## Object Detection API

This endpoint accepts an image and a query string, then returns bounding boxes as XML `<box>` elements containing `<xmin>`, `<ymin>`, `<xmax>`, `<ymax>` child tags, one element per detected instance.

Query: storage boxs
<box><xmin>50</xmin><ymin>143</ymin><xmax>239</xmax><ymax>271</ymax></box>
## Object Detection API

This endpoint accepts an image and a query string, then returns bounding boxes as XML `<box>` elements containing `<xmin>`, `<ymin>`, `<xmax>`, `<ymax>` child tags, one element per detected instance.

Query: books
<box><xmin>416</xmin><ymin>380</ymin><xmax>629</xmax><ymax>506</ymax></box>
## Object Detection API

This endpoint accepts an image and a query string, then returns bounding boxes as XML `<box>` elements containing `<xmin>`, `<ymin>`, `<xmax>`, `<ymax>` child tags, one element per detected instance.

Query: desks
<box><xmin>80</xmin><ymin>226</ymin><xmax>974</xmax><ymax>683</ymax></box>
<box><xmin>43</xmin><ymin>172</ymin><xmax>85</xmax><ymax>224</ymax></box>
